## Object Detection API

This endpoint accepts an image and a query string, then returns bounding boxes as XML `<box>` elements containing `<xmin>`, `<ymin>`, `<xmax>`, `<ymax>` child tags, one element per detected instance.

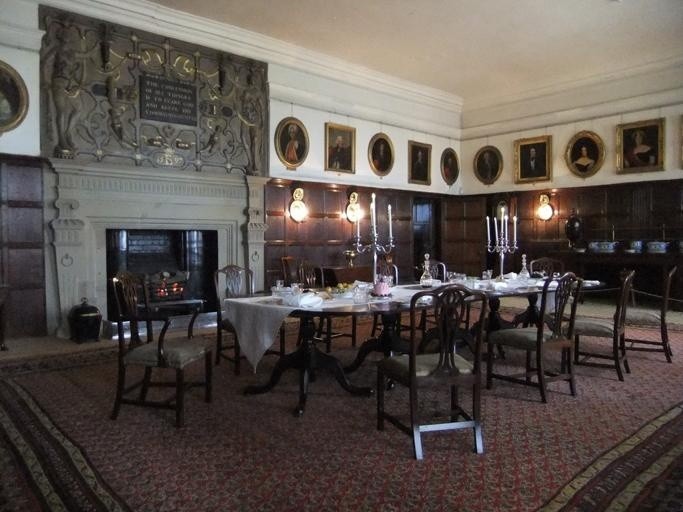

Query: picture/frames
<box><xmin>275</xmin><ymin>117</ymin><xmax>310</xmax><ymax>170</ymax></box>
<box><xmin>615</xmin><ymin>117</ymin><xmax>667</xmax><ymax>174</ymax></box>
<box><xmin>565</xmin><ymin>129</ymin><xmax>605</xmax><ymax>179</ymax></box>
<box><xmin>514</xmin><ymin>136</ymin><xmax>553</xmax><ymax>184</ymax></box>
<box><xmin>368</xmin><ymin>132</ymin><xmax>395</xmax><ymax>177</ymax></box>
<box><xmin>440</xmin><ymin>148</ymin><xmax>461</xmax><ymax>185</ymax></box>
<box><xmin>407</xmin><ymin>140</ymin><xmax>433</xmax><ymax>185</ymax></box>
<box><xmin>325</xmin><ymin>122</ymin><xmax>356</xmax><ymax>173</ymax></box>
<box><xmin>0</xmin><ymin>59</ymin><xmax>29</xmax><ymax>134</ymax></box>
<box><xmin>473</xmin><ymin>145</ymin><xmax>504</xmax><ymax>186</ymax></box>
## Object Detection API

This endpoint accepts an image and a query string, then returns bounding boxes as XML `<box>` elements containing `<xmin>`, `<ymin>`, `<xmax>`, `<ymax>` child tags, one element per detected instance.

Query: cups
<box><xmin>276</xmin><ymin>279</ymin><xmax>284</xmax><ymax>286</ymax></box>
<box><xmin>488</xmin><ymin>269</ymin><xmax>493</xmax><ymax>277</ymax></box>
<box><xmin>447</xmin><ymin>272</ymin><xmax>455</xmax><ymax>279</ymax></box>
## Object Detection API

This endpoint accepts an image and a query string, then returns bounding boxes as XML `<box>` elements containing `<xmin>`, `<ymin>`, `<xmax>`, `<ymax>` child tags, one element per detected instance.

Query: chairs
<box><xmin>297</xmin><ymin>263</ymin><xmax>356</xmax><ymax>354</ymax></box>
<box><xmin>215</xmin><ymin>265</ymin><xmax>286</xmax><ymax>374</ymax></box>
<box><xmin>376</xmin><ymin>285</ymin><xmax>487</xmax><ymax>461</ymax></box>
<box><xmin>620</xmin><ymin>265</ymin><xmax>677</xmax><ymax>363</ymax></box>
<box><xmin>108</xmin><ymin>268</ymin><xmax>213</xmax><ymax>428</ymax></box>
<box><xmin>488</xmin><ymin>272</ymin><xmax>583</xmax><ymax>403</ymax></box>
<box><xmin>372</xmin><ymin>262</ymin><xmax>413</xmax><ymax>339</ymax></box>
<box><xmin>562</xmin><ymin>268</ymin><xmax>630</xmax><ymax>381</ymax></box>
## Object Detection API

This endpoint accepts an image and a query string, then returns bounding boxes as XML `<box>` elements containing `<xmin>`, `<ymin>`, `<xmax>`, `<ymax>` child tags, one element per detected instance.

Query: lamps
<box><xmin>290</xmin><ymin>200</ymin><xmax>309</xmax><ymax>222</ymax></box>
<box><xmin>538</xmin><ymin>205</ymin><xmax>553</xmax><ymax>221</ymax></box>
<box><xmin>346</xmin><ymin>206</ymin><xmax>364</xmax><ymax>222</ymax></box>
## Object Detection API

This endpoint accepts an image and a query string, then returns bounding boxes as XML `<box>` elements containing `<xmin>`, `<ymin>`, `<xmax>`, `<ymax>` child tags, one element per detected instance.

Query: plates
<box><xmin>329</xmin><ymin>293</ymin><xmax>344</xmax><ymax>299</ymax></box>
<box><xmin>302</xmin><ymin>296</ymin><xmax>322</xmax><ymax>307</ymax></box>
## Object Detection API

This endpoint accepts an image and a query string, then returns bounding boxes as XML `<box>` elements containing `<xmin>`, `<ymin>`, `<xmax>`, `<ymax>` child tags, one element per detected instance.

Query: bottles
<box><xmin>519</xmin><ymin>254</ymin><xmax>531</xmax><ymax>282</ymax></box>
<box><xmin>420</xmin><ymin>253</ymin><xmax>433</xmax><ymax>288</ymax></box>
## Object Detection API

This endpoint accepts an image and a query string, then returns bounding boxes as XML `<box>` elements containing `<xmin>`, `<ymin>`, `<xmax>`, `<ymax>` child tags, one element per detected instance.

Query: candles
<box><xmin>370</xmin><ymin>192</ymin><xmax>378</xmax><ymax>236</ymax></box>
<box><xmin>513</xmin><ymin>216</ymin><xmax>518</xmax><ymax>243</ymax></box>
<box><xmin>387</xmin><ymin>205</ymin><xmax>393</xmax><ymax>242</ymax></box>
<box><xmin>504</xmin><ymin>215</ymin><xmax>508</xmax><ymax>241</ymax></box>
<box><xmin>355</xmin><ymin>207</ymin><xmax>363</xmax><ymax>244</ymax></box>
<box><xmin>486</xmin><ymin>216</ymin><xmax>490</xmax><ymax>247</ymax></box>
<box><xmin>501</xmin><ymin>207</ymin><xmax>504</xmax><ymax>240</ymax></box>
<box><xmin>493</xmin><ymin>217</ymin><xmax>498</xmax><ymax>244</ymax></box>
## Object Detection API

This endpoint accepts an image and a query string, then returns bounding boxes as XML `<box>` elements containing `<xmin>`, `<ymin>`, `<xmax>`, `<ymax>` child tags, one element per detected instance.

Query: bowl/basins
<box><xmin>598</xmin><ymin>240</ymin><xmax>620</xmax><ymax>249</ymax></box>
<box><xmin>631</xmin><ymin>240</ymin><xmax>642</xmax><ymax>249</ymax></box>
<box><xmin>647</xmin><ymin>241</ymin><xmax>667</xmax><ymax>251</ymax></box>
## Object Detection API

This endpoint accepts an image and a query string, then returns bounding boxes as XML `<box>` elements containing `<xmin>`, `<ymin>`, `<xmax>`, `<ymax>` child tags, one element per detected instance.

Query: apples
<box><xmin>325</xmin><ymin>282</ymin><xmax>351</xmax><ymax>294</ymax></box>
